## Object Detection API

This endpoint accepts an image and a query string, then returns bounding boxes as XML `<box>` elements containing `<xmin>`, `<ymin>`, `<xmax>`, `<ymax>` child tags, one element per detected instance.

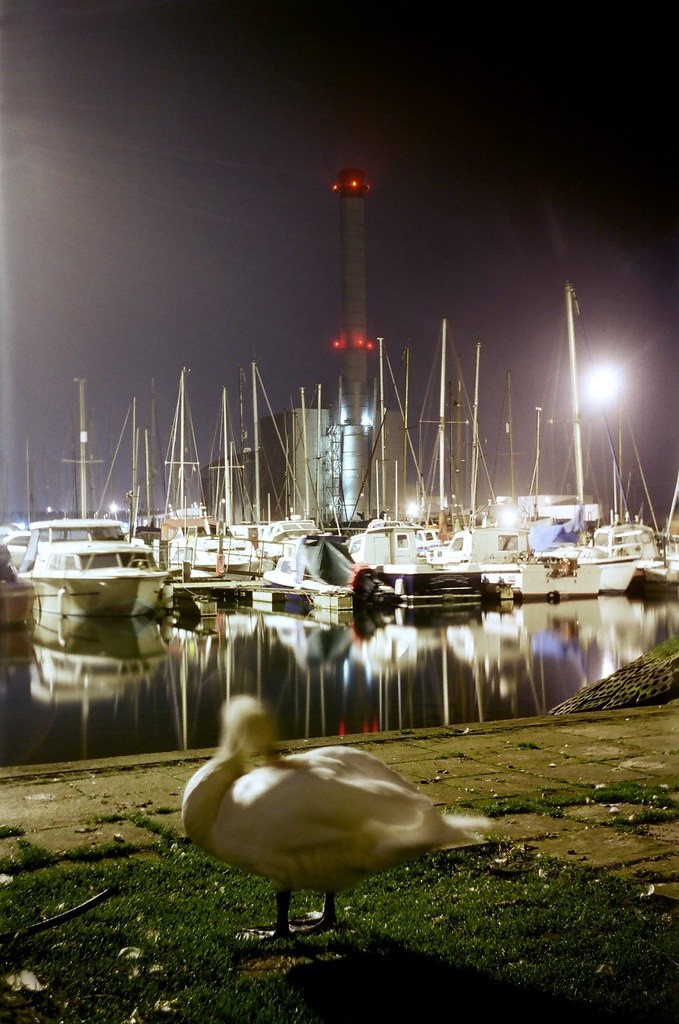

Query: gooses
<box><xmin>181</xmin><ymin>694</ymin><xmax>496</xmax><ymax>942</ymax></box>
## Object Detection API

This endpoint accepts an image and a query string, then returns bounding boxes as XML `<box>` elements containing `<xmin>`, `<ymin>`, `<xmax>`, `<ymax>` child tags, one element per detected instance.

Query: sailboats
<box><xmin>0</xmin><ymin>280</ymin><xmax>679</xmax><ymax>619</ymax></box>
<box><xmin>28</xmin><ymin>608</ymin><xmax>169</xmax><ymax>760</ymax></box>
